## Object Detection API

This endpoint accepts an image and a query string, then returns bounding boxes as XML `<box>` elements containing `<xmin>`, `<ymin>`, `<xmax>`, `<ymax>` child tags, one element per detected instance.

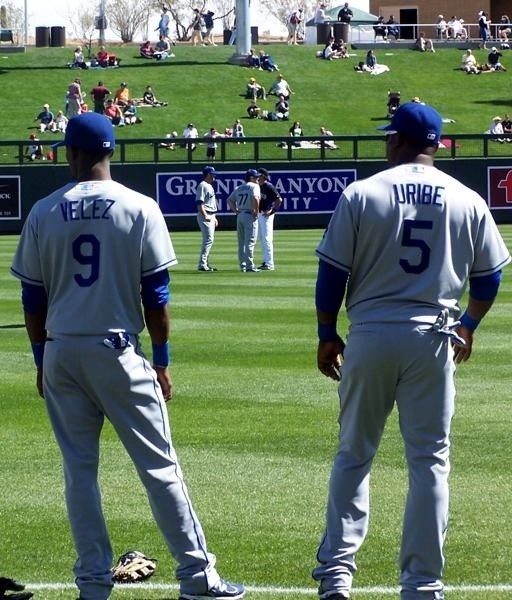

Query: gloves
<box><xmin>432</xmin><ymin>308</ymin><xmax>466</xmax><ymax>346</ymax></box>
<box><xmin>103</xmin><ymin>332</ymin><xmax>129</xmax><ymax>348</ymax></box>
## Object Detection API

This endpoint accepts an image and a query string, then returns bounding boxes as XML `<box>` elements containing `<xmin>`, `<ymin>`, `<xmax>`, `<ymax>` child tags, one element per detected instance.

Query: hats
<box><xmin>203</xmin><ymin>166</ymin><xmax>219</xmax><ymax>175</ymax></box>
<box><xmin>50</xmin><ymin>112</ymin><xmax>115</xmax><ymax>152</ymax></box>
<box><xmin>244</xmin><ymin>168</ymin><xmax>263</xmax><ymax>177</ymax></box>
<box><xmin>377</xmin><ymin>103</ymin><xmax>443</xmax><ymax>144</ymax></box>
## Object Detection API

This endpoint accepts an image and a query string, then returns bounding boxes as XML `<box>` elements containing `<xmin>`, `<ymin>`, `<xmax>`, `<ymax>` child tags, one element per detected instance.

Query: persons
<box><xmin>491</xmin><ymin>114</ymin><xmax>511</xmax><ymax>144</ymax></box>
<box><xmin>25</xmin><ymin>7</ymin><xmax>247</xmax><ymax>164</ymax></box>
<box><xmin>227</xmin><ymin>168</ymin><xmax>262</xmax><ymax>273</ymax></box>
<box><xmin>283</xmin><ymin>121</ymin><xmax>339</xmax><ymax>149</ymax></box>
<box><xmin>196</xmin><ymin>166</ymin><xmax>219</xmax><ymax>272</ymax></box>
<box><xmin>258</xmin><ymin>167</ymin><xmax>282</xmax><ymax>269</ymax></box>
<box><xmin>233</xmin><ymin>48</ymin><xmax>293</xmax><ymax>143</ymax></box>
<box><xmin>287</xmin><ymin>2</ymin><xmax>512</xmax><ymax>74</ymax></box>
<box><xmin>315</xmin><ymin>101</ymin><xmax>512</xmax><ymax>599</ymax></box>
<box><xmin>9</xmin><ymin>112</ymin><xmax>247</xmax><ymax>600</ymax></box>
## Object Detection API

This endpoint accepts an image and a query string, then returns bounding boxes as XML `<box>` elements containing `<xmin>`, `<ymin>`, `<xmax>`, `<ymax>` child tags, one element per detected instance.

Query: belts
<box><xmin>238</xmin><ymin>211</ymin><xmax>252</xmax><ymax>214</ymax></box>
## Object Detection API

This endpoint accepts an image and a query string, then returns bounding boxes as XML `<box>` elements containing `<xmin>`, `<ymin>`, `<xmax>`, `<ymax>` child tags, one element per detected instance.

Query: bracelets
<box><xmin>459</xmin><ymin>312</ymin><xmax>479</xmax><ymax>332</ymax></box>
<box><xmin>153</xmin><ymin>341</ymin><xmax>170</xmax><ymax>367</ymax></box>
<box><xmin>319</xmin><ymin>322</ymin><xmax>337</xmax><ymax>341</ymax></box>
<box><xmin>28</xmin><ymin>343</ymin><xmax>47</xmax><ymax>373</ymax></box>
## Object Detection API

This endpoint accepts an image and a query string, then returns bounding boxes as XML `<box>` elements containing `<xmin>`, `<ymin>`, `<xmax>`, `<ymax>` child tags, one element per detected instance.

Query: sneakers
<box><xmin>257</xmin><ymin>263</ymin><xmax>269</xmax><ymax>270</ymax></box>
<box><xmin>246</xmin><ymin>268</ymin><xmax>262</xmax><ymax>272</ymax></box>
<box><xmin>178</xmin><ymin>579</ymin><xmax>246</xmax><ymax>600</ymax></box>
<box><xmin>199</xmin><ymin>267</ymin><xmax>214</xmax><ymax>271</ymax></box>
